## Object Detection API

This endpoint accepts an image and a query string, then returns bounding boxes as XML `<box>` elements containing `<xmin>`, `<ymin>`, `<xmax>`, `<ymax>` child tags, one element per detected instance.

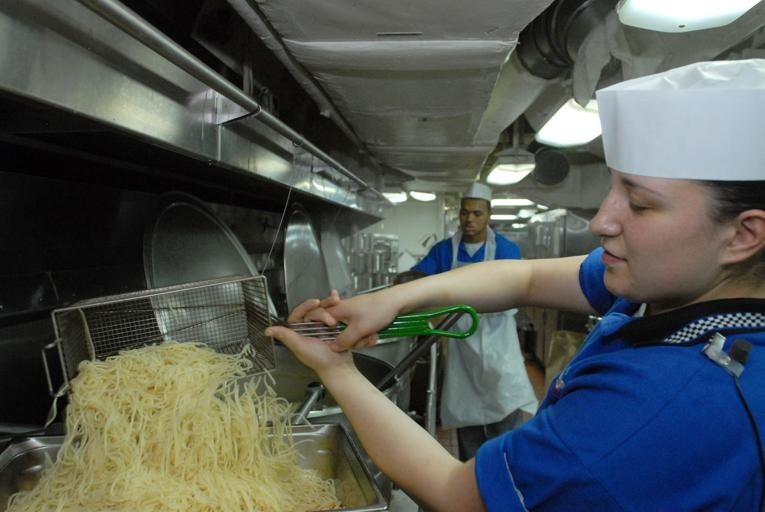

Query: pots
<box><xmin>221</xmin><ymin>348</ymin><xmax>402</xmax><ymax>507</ymax></box>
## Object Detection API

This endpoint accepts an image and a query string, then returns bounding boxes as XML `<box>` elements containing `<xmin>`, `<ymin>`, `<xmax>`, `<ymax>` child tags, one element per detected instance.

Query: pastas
<box><xmin>5</xmin><ymin>340</ymin><xmax>343</xmax><ymax>512</ymax></box>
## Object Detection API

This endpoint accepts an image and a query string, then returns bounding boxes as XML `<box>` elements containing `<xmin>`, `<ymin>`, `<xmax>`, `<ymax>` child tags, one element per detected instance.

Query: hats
<box><xmin>461</xmin><ymin>182</ymin><xmax>492</xmax><ymax>203</ymax></box>
<box><xmin>594</xmin><ymin>59</ymin><xmax>765</xmax><ymax>182</ymax></box>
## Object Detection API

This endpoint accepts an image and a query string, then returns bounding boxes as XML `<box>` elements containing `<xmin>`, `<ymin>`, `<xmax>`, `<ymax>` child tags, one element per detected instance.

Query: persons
<box><xmin>264</xmin><ymin>61</ymin><xmax>765</xmax><ymax>512</ymax></box>
<box><xmin>407</xmin><ymin>181</ymin><xmax>540</xmax><ymax>464</ymax></box>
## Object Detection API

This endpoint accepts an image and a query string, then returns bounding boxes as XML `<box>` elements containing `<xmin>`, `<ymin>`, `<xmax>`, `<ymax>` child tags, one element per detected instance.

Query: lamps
<box><xmin>479</xmin><ymin>118</ymin><xmax>537</xmax><ymax>188</ymax></box>
<box><xmin>614</xmin><ymin>0</ymin><xmax>763</xmax><ymax>33</ymax></box>
<box><xmin>382</xmin><ymin>186</ymin><xmax>407</xmax><ymax>203</ymax></box>
<box><xmin>491</xmin><ymin>192</ymin><xmax>534</xmax><ymax>208</ymax></box>
<box><xmin>524</xmin><ymin>77</ymin><xmax>603</xmax><ymax>149</ymax></box>
